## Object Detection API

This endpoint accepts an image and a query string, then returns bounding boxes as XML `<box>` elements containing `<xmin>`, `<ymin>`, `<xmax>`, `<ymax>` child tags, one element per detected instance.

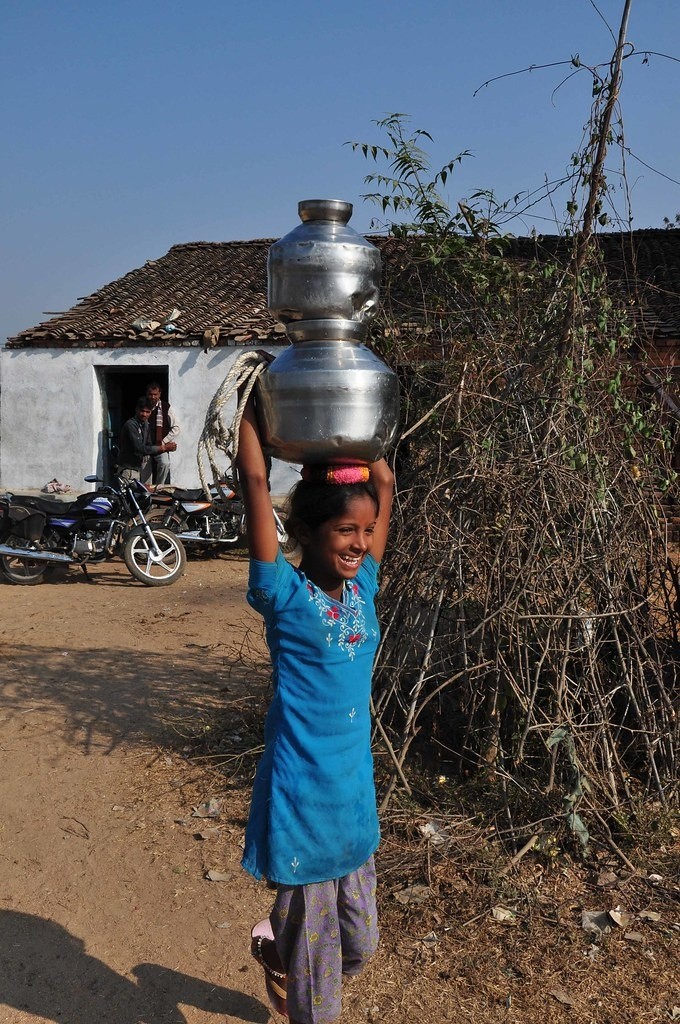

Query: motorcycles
<box><xmin>121</xmin><ymin>463</ymin><xmax>301</xmax><ymax>566</ymax></box>
<box><xmin>0</xmin><ymin>443</ymin><xmax>186</xmax><ymax>586</ymax></box>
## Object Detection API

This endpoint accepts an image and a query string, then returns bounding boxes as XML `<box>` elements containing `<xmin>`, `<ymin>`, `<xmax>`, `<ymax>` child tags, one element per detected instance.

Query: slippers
<box><xmin>251</xmin><ymin>917</ymin><xmax>289</xmax><ymax>1018</ymax></box>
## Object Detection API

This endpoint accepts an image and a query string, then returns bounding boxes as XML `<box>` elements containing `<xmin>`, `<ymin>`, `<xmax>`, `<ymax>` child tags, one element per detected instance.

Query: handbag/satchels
<box><xmin>10</xmin><ymin>504</ymin><xmax>47</xmax><ymax>541</ymax></box>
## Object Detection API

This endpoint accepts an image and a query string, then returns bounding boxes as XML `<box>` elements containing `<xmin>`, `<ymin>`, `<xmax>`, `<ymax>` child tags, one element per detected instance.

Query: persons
<box><xmin>116</xmin><ymin>382</ymin><xmax>177</xmax><ymax>484</ymax></box>
<box><xmin>236</xmin><ymin>349</ymin><xmax>395</xmax><ymax>1024</ymax></box>
